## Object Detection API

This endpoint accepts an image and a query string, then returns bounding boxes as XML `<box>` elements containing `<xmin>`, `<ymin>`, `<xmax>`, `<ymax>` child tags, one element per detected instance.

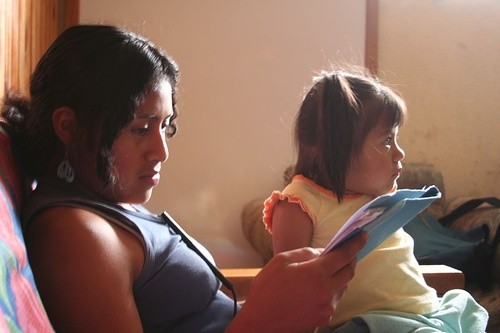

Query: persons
<box><xmin>1</xmin><ymin>24</ymin><xmax>369</xmax><ymax>332</ymax></box>
<box><xmin>263</xmin><ymin>71</ymin><xmax>489</xmax><ymax>333</ymax></box>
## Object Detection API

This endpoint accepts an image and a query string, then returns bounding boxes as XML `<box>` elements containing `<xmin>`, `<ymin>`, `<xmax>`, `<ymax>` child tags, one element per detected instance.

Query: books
<box><xmin>317</xmin><ymin>186</ymin><xmax>443</xmax><ymax>261</ymax></box>
